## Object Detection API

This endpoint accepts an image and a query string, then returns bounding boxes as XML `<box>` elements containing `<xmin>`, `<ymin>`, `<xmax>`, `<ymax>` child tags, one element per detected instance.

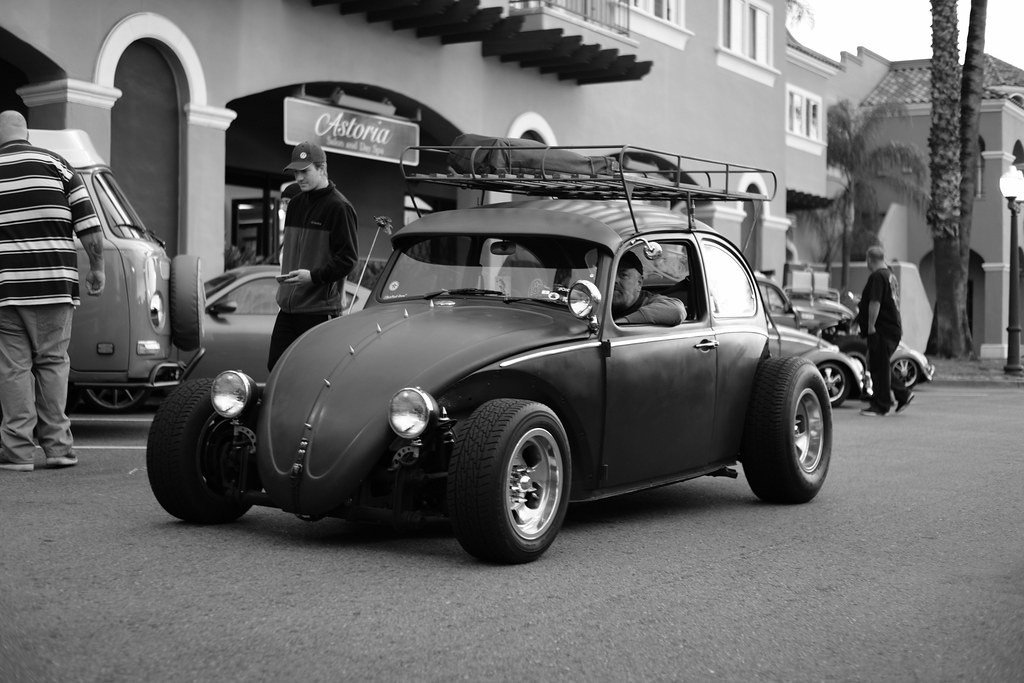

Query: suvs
<box><xmin>31</xmin><ymin>128</ymin><xmax>206</xmax><ymax>419</ymax></box>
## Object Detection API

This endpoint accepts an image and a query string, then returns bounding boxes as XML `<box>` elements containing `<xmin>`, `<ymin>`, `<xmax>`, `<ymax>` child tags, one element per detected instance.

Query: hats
<box><xmin>619</xmin><ymin>251</ymin><xmax>643</xmax><ymax>276</ymax></box>
<box><xmin>283</xmin><ymin>140</ymin><xmax>327</xmax><ymax>171</ymax></box>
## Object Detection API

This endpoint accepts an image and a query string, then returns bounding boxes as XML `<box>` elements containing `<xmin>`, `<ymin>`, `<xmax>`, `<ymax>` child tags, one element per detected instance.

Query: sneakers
<box><xmin>0</xmin><ymin>449</ymin><xmax>36</xmax><ymax>472</ymax></box>
<box><xmin>895</xmin><ymin>390</ymin><xmax>914</xmax><ymax>414</ymax></box>
<box><xmin>860</xmin><ymin>404</ymin><xmax>890</xmax><ymax>417</ymax></box>
<box><xmin>45</xmin><ymin>449</ymin><xmax>78</xmax><ymax>465</ymax></box>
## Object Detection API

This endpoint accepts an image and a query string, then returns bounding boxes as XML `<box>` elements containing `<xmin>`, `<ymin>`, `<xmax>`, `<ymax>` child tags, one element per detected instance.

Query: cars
<box><xmin>138</xmin><ymin>146</ymin><xmax>836</xmax><ymax>568</ymax></box>
<box><xmin>755</xmin><ymin>278</ymin><xmax>935</xmax><ymax>411</ymax></box>
<box><xmin>82</xmin><ymin>267</ymin><xmax>376</xmax><ymax>414</ymax></box>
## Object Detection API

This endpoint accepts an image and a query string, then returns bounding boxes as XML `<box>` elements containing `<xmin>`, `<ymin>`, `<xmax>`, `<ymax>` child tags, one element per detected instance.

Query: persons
<box><xmin>267</xmin><ymin>141</ymin><xmax>360</xmax><ymax>372</ymax></box>
<box><xmin>612</xmin><ymin>250</ymin><xmax>687</xmax><ymax>327</ymax></box>
<box><xmin>857</xmin><ymin>246</ymin><xmax>915</xmax><ymax>417</ymax></box>
<box><xmin>0</xmin><ymin>109</ymin><xmax>106</xmax><ymax>472</ymax></box>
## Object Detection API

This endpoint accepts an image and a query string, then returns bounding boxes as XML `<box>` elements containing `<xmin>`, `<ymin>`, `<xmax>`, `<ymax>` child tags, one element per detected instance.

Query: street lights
<box><xmin>997</xmin><ymin>165</ymin><xmax>1023</xmax><ymax>375</ymax></box>
